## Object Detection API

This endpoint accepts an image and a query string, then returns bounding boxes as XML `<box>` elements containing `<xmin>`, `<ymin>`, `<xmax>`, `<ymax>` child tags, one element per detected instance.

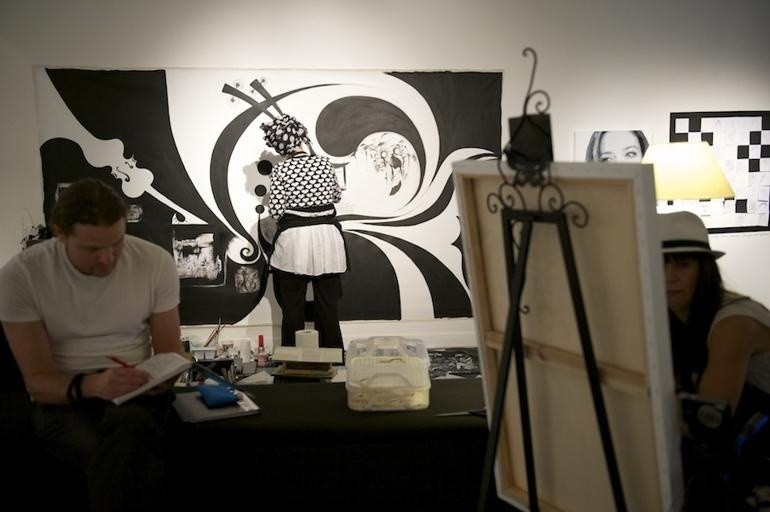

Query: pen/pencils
<box><xmin>205</xmin><ymin>318</ymin><xmax>225</xmax><ymax>348</ymax></box>
<box><xmin>107</xmin><ymin>356</ymin><xmax>132</xmax><ymax>369</ymax></box>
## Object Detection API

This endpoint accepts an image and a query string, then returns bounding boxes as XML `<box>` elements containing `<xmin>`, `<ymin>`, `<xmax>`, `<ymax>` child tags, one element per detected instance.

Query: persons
<box><xmin>266</xmin><ymin>115</ymin><xmax>346</xmax><ymax>349</ymax></box>
<box><xmin>661</xmin><ymin>212</ymin><xmax>770</xmax><ymax>416</ymax></box>
<box><xmin>585</xmin><ymin>131</ymin><xmax>649</xmax><ymax>165</ymax></box>
<box><xmin>0</xmin><ymin>178</ymin><xmax>190</xmax><ymax>406</ymax></box>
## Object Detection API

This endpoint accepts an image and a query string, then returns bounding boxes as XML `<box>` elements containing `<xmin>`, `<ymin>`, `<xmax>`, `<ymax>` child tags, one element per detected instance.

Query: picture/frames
<box><xmin>450</xmin><ymin>158</ymin><xmax>685</xmax><ymax>511</ymax></box>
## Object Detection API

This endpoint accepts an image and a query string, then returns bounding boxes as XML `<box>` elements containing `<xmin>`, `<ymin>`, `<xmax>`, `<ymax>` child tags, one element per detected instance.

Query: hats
<box><xmin>654</xmin><ymin>210</ymin><xmax>726</xmax><ymax>260</ymax></box>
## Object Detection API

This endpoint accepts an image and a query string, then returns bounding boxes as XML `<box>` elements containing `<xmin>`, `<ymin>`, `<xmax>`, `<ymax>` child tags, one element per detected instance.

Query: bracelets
<box><xmin>67</xmin><ymin>373</ymin><xmax>88</xmax><ymax>404</ymax></box>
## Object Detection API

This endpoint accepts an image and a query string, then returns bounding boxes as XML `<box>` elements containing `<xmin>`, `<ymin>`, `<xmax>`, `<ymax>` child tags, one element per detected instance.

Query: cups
<box><xmin>182</xmin><ymin>335</ymin><xmax>190</xmax><ymax>354</ymax></box>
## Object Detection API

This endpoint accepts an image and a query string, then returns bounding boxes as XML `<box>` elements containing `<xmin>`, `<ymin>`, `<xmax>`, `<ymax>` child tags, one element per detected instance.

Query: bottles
<box><xmin>254</xmin><ymin>335</ymin><xmax>269</xmax><ymax>368</ymax></box>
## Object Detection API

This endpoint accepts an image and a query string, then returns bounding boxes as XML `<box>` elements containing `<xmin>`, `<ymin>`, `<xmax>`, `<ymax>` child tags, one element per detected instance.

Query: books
<box><xmin>173</xmin><ymin>389</ymin><xmax>261</xmax><ymax>422</ymax></box>
<box><xmin>111</xmin><ymin>352</ymin><xmax>192</xmax><ymax>406</ymax></box>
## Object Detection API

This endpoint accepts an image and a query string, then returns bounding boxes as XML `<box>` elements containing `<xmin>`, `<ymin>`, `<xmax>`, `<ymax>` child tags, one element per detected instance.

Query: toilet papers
<box><xmin>294</xmin><ymin>329</ymin><xmax>320</xmax><ymax>350</ymax></box>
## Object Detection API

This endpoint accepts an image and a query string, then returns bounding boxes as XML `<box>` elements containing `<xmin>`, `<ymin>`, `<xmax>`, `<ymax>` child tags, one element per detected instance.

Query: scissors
<box><xmin>433</xmin><ymin>408</ymin><xmax>486</xmax><ymax>418</ymax></box>
